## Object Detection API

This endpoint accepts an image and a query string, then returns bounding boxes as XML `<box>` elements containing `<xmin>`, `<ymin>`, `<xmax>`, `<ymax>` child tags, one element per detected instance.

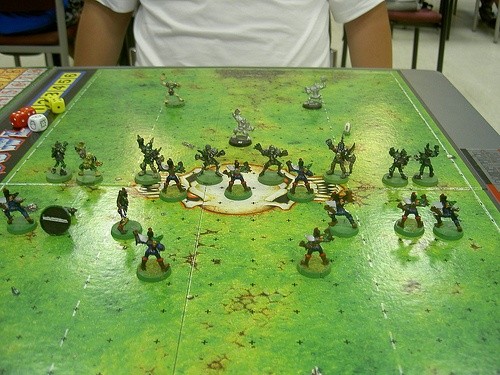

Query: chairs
<box><xmin>341</xmin><ymin>0</ymin><xmax>453</xmax><ymax>73</ymax></box>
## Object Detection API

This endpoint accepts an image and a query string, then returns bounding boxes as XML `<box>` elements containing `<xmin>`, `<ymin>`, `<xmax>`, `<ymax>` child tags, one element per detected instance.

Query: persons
<box><xmin>73</xmin><ymin>0</ymin><xmax>392</xmax><ymax>69</ymax></box>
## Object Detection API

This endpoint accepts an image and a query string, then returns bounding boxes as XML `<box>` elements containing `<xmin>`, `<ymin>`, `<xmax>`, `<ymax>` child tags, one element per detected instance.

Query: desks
<box><xmin>0</xmin><ymin>66</ymin><xmax>500</xmax><ymax>375</ymax></box>
